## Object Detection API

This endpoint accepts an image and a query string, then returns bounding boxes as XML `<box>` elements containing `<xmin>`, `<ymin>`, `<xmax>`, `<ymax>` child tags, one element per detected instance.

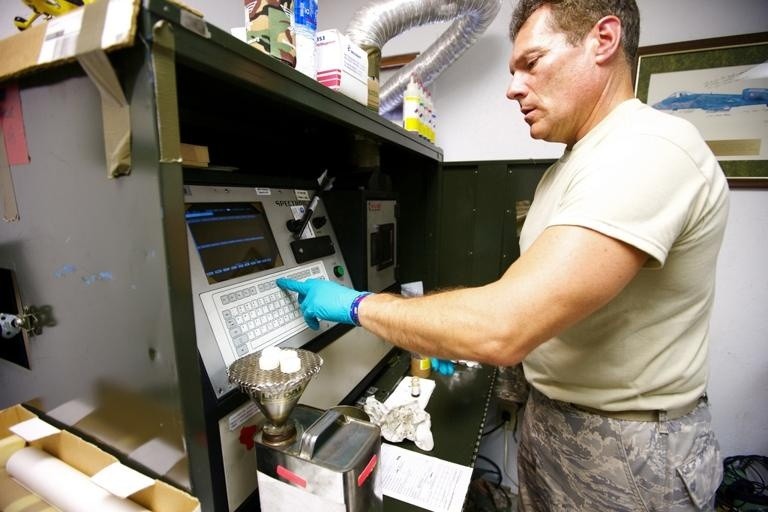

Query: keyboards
<box><xmin>198</xmin><ymin>259</ymin><xmax>330</xmax><ymax>368</ymax></box>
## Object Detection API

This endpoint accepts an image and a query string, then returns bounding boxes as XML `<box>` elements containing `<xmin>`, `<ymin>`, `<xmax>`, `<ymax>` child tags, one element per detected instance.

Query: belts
<box><xmin>571</xmin><ymin>395</ymin><xmax>705</xmax><ymax>424</ymax></box>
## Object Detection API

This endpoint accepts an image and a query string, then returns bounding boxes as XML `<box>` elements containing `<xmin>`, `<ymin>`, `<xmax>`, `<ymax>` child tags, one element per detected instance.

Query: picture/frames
<box><xmin>624</xmin><ymin>32</ymin><xmax>768</xmax><ymax>193</ymax></box>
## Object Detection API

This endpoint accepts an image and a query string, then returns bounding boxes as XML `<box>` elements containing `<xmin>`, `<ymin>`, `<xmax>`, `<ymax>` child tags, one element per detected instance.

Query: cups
<box><xmin>410</xmin><ymin>350</ymin><xmax>432</xmax><ymax>379</ymax></box>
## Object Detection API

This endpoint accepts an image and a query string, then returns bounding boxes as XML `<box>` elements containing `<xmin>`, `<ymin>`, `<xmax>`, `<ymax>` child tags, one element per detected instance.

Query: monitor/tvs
<box><xmin>180</xmin><ymin>198</ymin><xmax>286</xmax><ymax>287</ymax></box>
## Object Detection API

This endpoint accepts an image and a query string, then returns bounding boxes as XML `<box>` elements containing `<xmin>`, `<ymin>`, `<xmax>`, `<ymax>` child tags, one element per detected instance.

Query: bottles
<box><xmin>292</xmin><ymin>0</ymin><xmax>321</xmax><ymax>80</ymax></box>
<box><xmin>243</xmin><ymin>0</ymin><xmax>297</xmax><ymax>69</ymax></box>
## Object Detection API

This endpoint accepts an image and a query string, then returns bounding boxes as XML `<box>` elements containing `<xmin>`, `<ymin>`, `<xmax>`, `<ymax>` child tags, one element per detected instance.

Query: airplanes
<box><xmin>646</xmin><ymin>87</ymin><xmax>767</xmax><ymax>114</ymax></box>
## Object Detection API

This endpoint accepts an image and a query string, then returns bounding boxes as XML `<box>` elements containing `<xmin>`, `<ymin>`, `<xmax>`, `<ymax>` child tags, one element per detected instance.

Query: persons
<box><xmin>274</xmin><ymin>0</ymin><xmax>731</xmax><ymax>512</ymax></box>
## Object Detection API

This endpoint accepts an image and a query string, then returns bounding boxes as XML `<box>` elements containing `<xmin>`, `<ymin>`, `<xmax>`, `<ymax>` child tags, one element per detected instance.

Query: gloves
<box><xmin>276</xmin><ymin>277</ymin><xmax>374</xmax><ymax>332</ymax></box>
